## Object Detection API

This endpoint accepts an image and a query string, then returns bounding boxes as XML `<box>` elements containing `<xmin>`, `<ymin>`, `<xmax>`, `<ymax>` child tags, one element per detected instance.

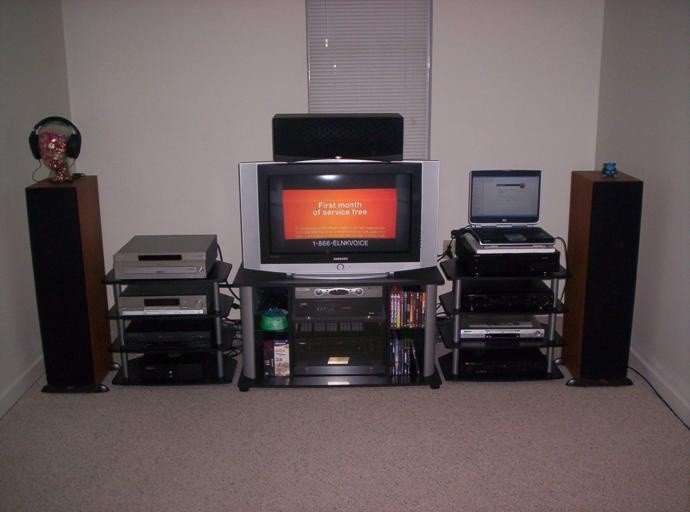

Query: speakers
<box><xmin>552</xmin><ymin>171</ymin><xmax>643</xmax><ymax>387</ymax></box>
<box><xmin>25</xmin><ymin>175</ymin><xmax>121</xmax><ymax>393</ymax></box>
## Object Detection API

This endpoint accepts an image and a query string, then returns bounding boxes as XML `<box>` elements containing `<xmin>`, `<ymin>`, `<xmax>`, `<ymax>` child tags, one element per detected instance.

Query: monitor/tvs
<box><xmin>239</xmin><ymin>160</ymin><xmax>440</xmax><ymax>280</ymax></box>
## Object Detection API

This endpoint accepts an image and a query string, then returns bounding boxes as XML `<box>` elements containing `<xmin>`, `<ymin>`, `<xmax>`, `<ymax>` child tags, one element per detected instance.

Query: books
<box><xmin>387</xmin><ymin>287</ymin><xmax>428</xmax><ymax>376</ymax></box>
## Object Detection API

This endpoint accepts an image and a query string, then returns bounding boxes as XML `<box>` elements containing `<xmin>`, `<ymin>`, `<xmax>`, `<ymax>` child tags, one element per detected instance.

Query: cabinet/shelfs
<box><xmin>100</xmin><ymin>261</ymin><xmax>239</xmax><ymax>386</ymax></box>
<box><xmin>230</xmin><ymin>263</ymin><xmax>449</xmax><ymax>391</ymax></box>
<box><xmin>438</xmin><ymin>255</ymin><xmax>573</xmax><ymax>383</ymax></box>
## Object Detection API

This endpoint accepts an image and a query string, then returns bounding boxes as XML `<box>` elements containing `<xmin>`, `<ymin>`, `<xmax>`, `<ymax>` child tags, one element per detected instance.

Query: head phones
<box><xmin>29</xmin><ymin>116</ymin><xmax>81</xmax><ymax>160</ymax></box>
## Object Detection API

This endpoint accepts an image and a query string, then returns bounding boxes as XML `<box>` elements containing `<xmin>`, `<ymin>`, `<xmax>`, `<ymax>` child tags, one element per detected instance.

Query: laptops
<box><xmin>468</xmin><ymin>170</ymin><xmax>555</xmax><ymax>246</ymax></box>
